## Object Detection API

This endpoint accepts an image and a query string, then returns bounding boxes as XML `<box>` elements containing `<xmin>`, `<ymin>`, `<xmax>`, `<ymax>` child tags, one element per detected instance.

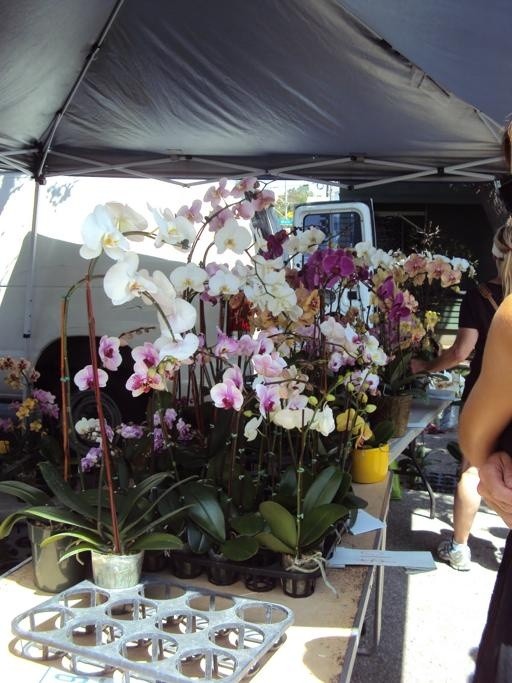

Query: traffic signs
<box><xmin>276</xmin><ymin>218</ymin><xmax>293</xmax><ymax>225</ymax></box>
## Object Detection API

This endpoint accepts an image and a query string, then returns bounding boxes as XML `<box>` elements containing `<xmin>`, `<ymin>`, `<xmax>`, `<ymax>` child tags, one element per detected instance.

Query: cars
<box><xmin>397</xmin><ymin>359</ymin><xmax>472</xmax><ymax>497</ymax></box>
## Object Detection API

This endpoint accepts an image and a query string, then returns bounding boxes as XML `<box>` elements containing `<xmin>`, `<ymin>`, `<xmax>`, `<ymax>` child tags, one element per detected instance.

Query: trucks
<box><xmin>329</xmin><ymin>174</ymin><xmax>509</xmax><ymax>353</ymax></box>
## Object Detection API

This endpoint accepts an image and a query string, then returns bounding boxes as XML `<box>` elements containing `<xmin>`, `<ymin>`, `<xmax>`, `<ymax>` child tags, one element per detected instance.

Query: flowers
<box><xmin>0</xmin><ymin>178</ymin><xmax>475</xmax><ymax>564</ymax></box>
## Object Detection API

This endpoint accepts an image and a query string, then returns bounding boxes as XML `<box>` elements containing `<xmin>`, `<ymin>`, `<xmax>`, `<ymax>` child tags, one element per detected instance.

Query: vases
<box><xmin>280</xmin><ymin>555</ymin><xmax>319</xmax><ymax>597</ymax></box>
<box><xmin>88</xmin><ymin>550</ymin><xmax>144</xmax><ymax>589</ymax></box>
<box><xmin>205</xmin><ymin>559</ymin><xmax>241</xmax><ymax>585</ymax></box>
<box><xmin>242</xmin><ymin>562</ymin><xmax>278</xmax><ymax>592</ymax></box>
<box><xmin>27</xmin><ymin>521</ymin><xmax>87</xmax><ymax>593</ymax></box>
<box><xmin>168</xmin><ymin>552</ymin><xmax>203</xmax><ymax>578</ymax></box>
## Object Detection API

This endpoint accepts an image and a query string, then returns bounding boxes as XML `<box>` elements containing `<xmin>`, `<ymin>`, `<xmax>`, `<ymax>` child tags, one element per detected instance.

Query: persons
<box><xmin>458</xmin><ymin>127</ymin><xmax>511</xmax><ymax>682</ymax></box>
<box><xmin>410</xmin><ymin>226</ymin><xmax>511</xmax><ymax>570</ymax></box>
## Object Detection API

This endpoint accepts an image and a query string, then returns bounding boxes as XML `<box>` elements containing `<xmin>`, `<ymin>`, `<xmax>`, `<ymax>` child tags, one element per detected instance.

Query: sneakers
<box><xmin>437</xmin><ymin>540</ymin><xmax>472</xmax><ymax>570</ymax></box>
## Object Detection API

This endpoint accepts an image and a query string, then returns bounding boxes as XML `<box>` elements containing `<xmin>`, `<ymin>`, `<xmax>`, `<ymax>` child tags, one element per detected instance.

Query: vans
<box><xmin>0</xmin><ymin>172</ymin><xmax>289</xmax><ymax>472</ymax></box>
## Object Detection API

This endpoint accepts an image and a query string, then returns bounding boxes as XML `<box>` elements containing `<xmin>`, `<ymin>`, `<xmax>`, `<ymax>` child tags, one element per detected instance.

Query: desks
<box><xmin>0</xmin><ymin>474</ymin><xmax>393</xmax><ymax>683</ymax></box>
